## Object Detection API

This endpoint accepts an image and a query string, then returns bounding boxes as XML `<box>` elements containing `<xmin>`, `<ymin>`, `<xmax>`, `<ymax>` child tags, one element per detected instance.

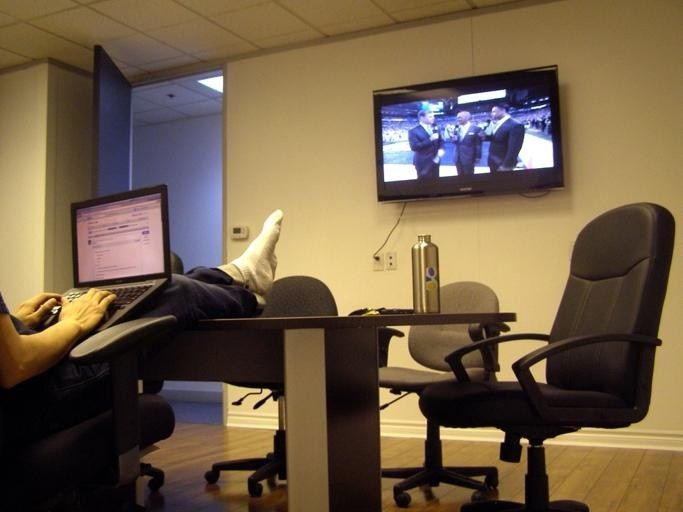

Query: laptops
<box><xmin>21</xmin><ymin>184</ymin><xmax>171</xmax><ymax>334</ymax></box>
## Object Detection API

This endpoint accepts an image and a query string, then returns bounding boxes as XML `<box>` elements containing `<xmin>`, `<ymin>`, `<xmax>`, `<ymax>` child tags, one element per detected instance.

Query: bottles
<box><xmin>411</xmin><ymin>235</ymin><xmax>440</xmax><ymax>314</ymax></box>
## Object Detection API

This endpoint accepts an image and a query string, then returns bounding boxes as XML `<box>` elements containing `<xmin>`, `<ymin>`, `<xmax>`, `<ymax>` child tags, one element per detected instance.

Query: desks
<box><xmin>204</xmin><ymin>311</ymin><xmax>517</xmax><ymax>509</ymax></box>
<box><xmin>145</xmin><ymin>311</ymin><xmax>517</xmax><ymax>512</ymax></box>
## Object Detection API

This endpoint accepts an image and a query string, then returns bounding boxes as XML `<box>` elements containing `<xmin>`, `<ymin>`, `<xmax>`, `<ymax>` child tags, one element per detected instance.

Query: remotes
<box><xmin>381</xmin><ymin>310</ymin><xmax>414</xmax><ymax>314</ymax></box>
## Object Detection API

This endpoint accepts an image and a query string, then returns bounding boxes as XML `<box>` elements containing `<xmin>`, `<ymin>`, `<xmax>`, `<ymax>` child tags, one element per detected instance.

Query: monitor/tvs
<box><xmin>372</xmin><ymin>64</ymin><xmax>565</xmax><ymax>204</ymax></box>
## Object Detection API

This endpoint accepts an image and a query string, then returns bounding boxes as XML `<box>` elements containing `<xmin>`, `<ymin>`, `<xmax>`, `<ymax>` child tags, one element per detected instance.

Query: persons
<box><xmin>382</xmin><ymin>103</ymin><xmax>552</xmax><ymax>180</ymax></box>
<box><xmin>0</xmin><ymin>208</ymin><xmax>285</xmax><ymax>456</ymax></box>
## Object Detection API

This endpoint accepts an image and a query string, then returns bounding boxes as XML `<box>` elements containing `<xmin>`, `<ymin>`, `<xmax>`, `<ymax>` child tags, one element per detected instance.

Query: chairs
<box><xmin>204</xmin><ymin>277</ymin><xmax>339</xmax><ymax>496</ymax></box>
<box><xmin>0</xmin><ymin>312</ymin><xmax>176</xmax><ymax>511</ymax></box>
<box><xmin>419</xmin><ymin>201</ymin><xmax>678</xmax><ymax>512</ymax></box>
<box><xmin>377</xmin><ymin>282</ymin><xmax>511</xmax><ymax>505</ymax></box>
